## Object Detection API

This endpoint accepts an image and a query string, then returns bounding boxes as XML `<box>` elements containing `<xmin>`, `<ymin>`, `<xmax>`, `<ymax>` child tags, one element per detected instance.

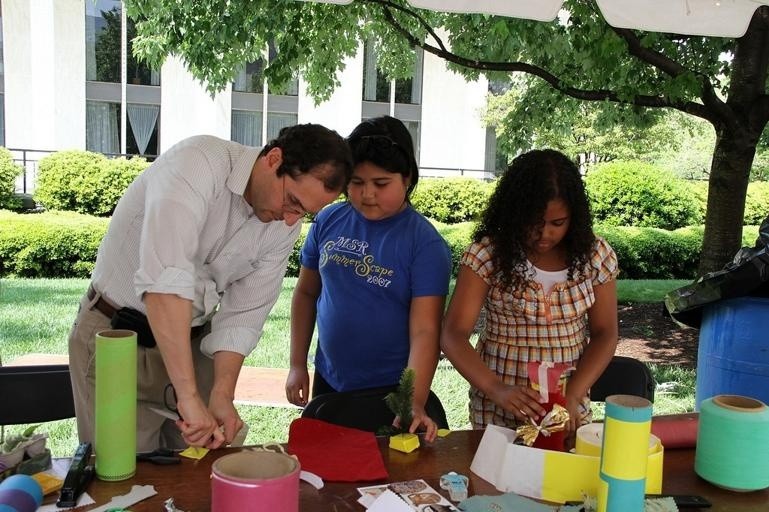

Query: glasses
<box><xmin>280</xmin><ymin>174</ymin><xmax>319</xmax><ymax>224</ymax></box>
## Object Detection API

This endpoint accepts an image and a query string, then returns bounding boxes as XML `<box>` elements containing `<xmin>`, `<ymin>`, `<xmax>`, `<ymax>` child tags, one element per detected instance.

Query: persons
<box><xmin>439</xmin><ymin>149</ymin><xmax>619</xmax><ymax>431</ymax></box>
<box><xmin>68</xmin><ymin>123</ymin><xmax>350</xmax><ymax>456</ymax></box>
<box><xmin>286</xmin><ymin>115</ymin><xmax>451</xmax><ymax>447</ymax></box>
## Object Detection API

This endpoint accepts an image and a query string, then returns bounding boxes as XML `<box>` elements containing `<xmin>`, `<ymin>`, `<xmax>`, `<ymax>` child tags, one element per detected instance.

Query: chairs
<box><xmin>590</xmin><ymin>355</ymin><xmax>655</xmax><ymax>416</ymax></box>
<box><xmin>0</xmin><ymin>363</ymin><xmax>77</xmax><ymax>428</ymax></box>
<box><xmin>300</xmin><ymin>387</ymin><xmax>448</xmax><ymax>432</ymax></box>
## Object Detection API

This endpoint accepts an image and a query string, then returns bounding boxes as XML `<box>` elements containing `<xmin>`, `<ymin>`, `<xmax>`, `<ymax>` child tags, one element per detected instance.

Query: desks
<box><xmin>1</xmin><ymin>419</ymin><xmax>768</xmax><ymax>512</ymax></box>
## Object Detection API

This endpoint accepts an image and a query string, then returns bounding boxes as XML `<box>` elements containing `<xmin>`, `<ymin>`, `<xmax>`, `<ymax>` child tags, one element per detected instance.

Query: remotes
<box><xmin>644</xmin><ymin>494</ymin><xmax>712</xmax><ymax>508</ymax></box>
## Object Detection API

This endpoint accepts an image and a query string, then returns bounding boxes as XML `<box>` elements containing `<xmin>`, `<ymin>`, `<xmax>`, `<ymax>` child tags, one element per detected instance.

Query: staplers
<box><xmin>56</xmin><ymin>442</ymin><xmax>96</xmax><ymax>507</ymax></box>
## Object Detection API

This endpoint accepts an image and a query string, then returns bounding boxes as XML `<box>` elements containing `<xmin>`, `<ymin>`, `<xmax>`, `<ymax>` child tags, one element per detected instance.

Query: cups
<box><xmin>208</xmin><ymin>450</ymin><xmax>302</xmax><ymax>511</ymax></box>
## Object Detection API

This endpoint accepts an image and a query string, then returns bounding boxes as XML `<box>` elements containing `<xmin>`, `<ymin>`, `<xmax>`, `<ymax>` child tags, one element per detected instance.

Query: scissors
<box><xmin>149</xmin><ymin>384</ymin><xmax>215</xmax><ymax>454</ymax></box>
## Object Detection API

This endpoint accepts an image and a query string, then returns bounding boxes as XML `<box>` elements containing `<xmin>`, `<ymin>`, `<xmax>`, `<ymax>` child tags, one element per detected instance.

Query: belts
<box><xmin>86</xmin><ymin>281</ymin><xmax>210</xmax><ymax>347</ymax></box>
